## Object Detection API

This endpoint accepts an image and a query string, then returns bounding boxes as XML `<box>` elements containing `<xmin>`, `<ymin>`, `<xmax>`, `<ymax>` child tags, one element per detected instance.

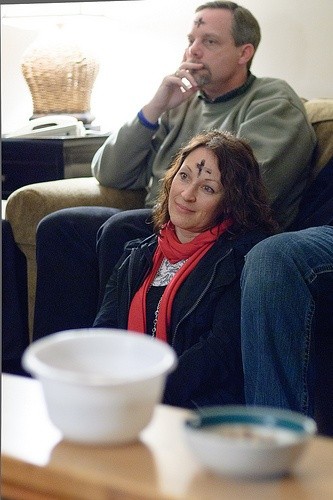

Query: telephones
<box><xmin>5</xmin><ymin>115</ymin><xmax>86</xmax><ymax>137</ymax></box>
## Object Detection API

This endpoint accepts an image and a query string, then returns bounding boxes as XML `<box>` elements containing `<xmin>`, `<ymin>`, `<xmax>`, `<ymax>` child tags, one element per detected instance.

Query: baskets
<box><xmin>25</xmin><ymin>61</ymin><xmax>100</xmax><ymax>120</ymax></box>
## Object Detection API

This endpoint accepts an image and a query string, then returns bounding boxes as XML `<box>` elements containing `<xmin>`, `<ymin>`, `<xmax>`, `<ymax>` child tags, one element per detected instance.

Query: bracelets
<box><xmin>138</xmin><ymin>110</ymin><xmax>159</xmax><ymax>129</ymax></box>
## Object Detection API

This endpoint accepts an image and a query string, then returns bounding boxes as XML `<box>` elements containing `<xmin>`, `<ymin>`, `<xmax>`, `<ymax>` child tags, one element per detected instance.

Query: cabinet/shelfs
<box><xmin>0</xmin><ymin>134</ymin><xmax>109</xmax><ymax>378</ymax></box>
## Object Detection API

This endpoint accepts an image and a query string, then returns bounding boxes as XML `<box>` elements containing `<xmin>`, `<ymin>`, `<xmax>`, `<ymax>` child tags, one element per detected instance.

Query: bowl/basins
<box><xmin>176</xmin><ymin>405</ymin><xmax>318</xmax><ymax>481</ymax></box>
<box><xmin>22</xmin><ymin>327</ymin><xmax>179</xmax><ymax>446</ymax></box>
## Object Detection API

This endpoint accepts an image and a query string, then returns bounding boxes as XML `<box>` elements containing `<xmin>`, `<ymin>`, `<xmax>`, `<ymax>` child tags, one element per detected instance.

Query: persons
<box><xmin>91</xmin><ymin>128</ymin><xmax>270</xmax><ymax>408</ymax></box>
<box><xmin>240</xmin><ymin>153</ymin><xmax>333</xmax><ymax>436</ymax></box>
<box><xmin>33</xmin><ymin>0</ymin><xmax>319</xmax><ymax>341</ymax></box>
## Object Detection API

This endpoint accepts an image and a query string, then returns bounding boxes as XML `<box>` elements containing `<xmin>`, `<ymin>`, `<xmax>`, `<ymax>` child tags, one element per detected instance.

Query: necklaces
<box><xmin>147</xmin><ymin>257</ymin><xmax>189</xmax><ymax>337</ymax></box>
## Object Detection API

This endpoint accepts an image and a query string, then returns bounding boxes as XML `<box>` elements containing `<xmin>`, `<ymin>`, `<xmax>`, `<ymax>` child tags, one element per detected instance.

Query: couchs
<box><xmin>5</xmin><ymin>97</ymin><xmax>333</xmax><ymax>347</ymax></box>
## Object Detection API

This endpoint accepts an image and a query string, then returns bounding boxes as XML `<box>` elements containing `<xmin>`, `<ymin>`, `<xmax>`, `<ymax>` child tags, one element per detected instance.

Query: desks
<box><xmin>0</xmin><ymin>371</ymin><xmax>333</xmax><ymax>500</ymax></box>
